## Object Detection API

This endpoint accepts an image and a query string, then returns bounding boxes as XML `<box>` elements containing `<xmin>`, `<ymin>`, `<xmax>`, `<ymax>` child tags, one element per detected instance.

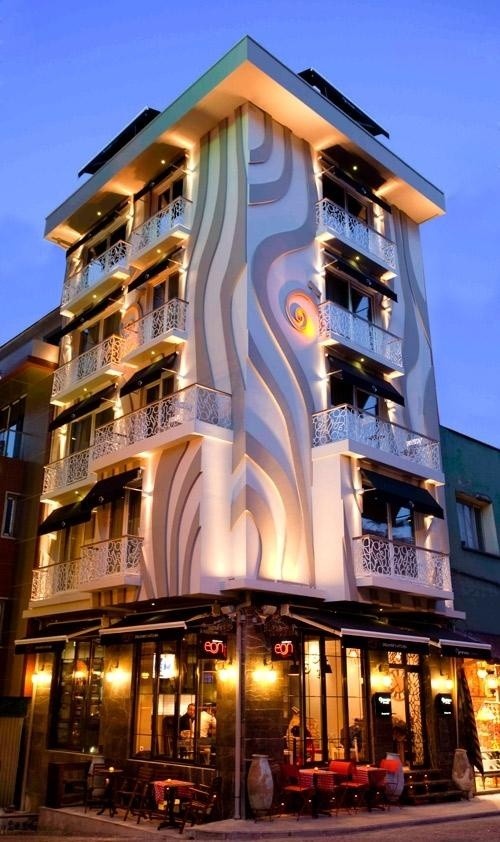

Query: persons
<box><xmin>179</xmin><ymin>702</ymin><xmax>195</xmax><ymax>751</ymax></box>
<box><xmin>192</xmin><ymin>702</ymin><xmax>216</xmax><ymax>764</ymax></box>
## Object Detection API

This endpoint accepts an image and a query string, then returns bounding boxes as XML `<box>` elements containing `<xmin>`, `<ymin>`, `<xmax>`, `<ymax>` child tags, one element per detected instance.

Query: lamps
<box><xmin>35</xmin><ymin>665</ymin><xmax>48</xmax><ymax>678</ymax></box>
<box><xmin>477</xmin><ymin>663</ymin><xmax>488</xmax><ymax>678</ymax></box>
<box><xmin>325</xmin><ymin>660</ymin><xmax>331</xmax><ymax>674</ymax></box>
<box><xmin>108</xmin><ymin>662</ymin><xmax>122</xmax><ymax>677</ymax></box>
<box><xmin>263</xmin><ymin>659</ymin><xmax>276</xmax><ymax>676</ymax></box>
<box><xmin>442</xmin><ymin>667</ymin><xmax>453</xmax><ymax>687</ymax></box>
<box><xmin>218</xmin><ymin>657</ymin><xmax>232</xmax><ymax>674</ymax></box>
<box><xmin>488</xmin><ymin>680</ymin><xmax>497</xmax><ymax>699</ymax></box>
<box><xmin>380</xmin><ymin>663</ymin><xmax>390</xmax><ymax>684</ymax></box>
<box><xmin>287</xmin><ymin>656</ymin><xmax>300</xmax><ymax>676</ymax></box>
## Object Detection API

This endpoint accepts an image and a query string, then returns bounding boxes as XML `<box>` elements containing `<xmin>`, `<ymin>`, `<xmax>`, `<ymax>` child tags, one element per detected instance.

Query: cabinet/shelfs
<box><xmin>47</xmin><ymin>762</ymin><xmax>91</xmax><ymax>809</ymax></box>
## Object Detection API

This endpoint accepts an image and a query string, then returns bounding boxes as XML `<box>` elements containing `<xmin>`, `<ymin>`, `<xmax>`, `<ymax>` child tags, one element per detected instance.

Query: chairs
<box><xmin>76</xmin><ymin>762</ymin><xmax>221</xmax><ymax>835</ymax></box>
<box><xmin>277</xmin><ymin>760</ymin><xmax>401</xmax><ymax>821</ymax></box>
